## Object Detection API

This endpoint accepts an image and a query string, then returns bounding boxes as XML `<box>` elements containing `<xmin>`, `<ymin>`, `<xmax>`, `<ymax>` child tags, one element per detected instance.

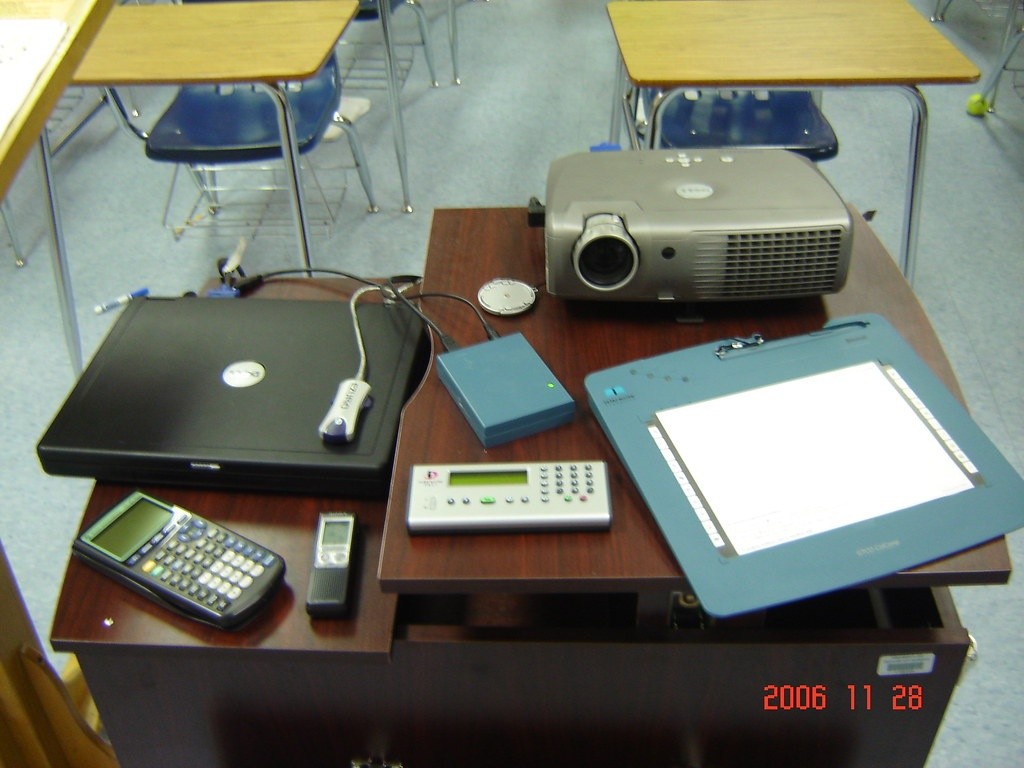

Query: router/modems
<box><xmin>436</xmin><ymin>332</ymin><xmax>576</xmax><ymax>448</ymax></box>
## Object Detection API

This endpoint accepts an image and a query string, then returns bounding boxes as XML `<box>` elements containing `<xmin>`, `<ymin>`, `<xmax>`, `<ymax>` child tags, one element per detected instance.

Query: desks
<box><xmin>604</xmin><ymin>1</ymin><xmax>983</xmax><ymax>294</ymax></box>
<box><xmin>339</xmin><ymin>0</ymin><xmax>442</xmax><ymax>92</ymax></box>
<box><xmin>68</xmin><ymin>0</ymin><xmax>360</xmax><ymax>280</ymax></box>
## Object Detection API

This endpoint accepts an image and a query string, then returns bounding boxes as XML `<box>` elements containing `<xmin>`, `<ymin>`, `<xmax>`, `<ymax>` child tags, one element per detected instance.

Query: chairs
<box><xmin>141</xmin><ymin>51</ymin><xmax>381</xmax><ymax>241</ymax></box>
<box><xmin>638</xmin><ymin>83</ymin><xmax>840</xmax><ymax>166</ymax></box>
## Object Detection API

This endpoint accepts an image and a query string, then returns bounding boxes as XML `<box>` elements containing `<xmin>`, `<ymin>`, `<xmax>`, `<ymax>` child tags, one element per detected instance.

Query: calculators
<box><xmin>71</xmin><ymin>488</ymin><xmax>288</xmax><ymax>632</ymax></box>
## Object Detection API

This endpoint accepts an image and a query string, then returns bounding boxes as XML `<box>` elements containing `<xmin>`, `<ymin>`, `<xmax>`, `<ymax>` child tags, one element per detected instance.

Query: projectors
<box><xmin>527</xmin><ymin>147</ymin><xmax>854</xmax><ymax>303</ymax></box>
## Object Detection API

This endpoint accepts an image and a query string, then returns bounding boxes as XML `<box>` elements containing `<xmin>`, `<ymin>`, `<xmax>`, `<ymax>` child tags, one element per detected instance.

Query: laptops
<box><xmin>36</xmin><ymin>295</ymin><xmax>424</xmax><ymax>498</ymax></box>
